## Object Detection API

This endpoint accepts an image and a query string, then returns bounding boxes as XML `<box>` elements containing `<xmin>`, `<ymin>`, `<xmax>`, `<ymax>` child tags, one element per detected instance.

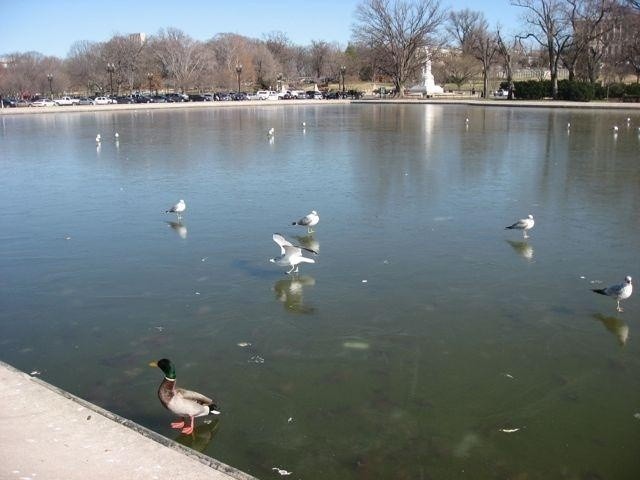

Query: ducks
<box><xmin>175</xmin><ymin>420</ymin><xmax>219</xmax><ymax>452</ymax></box>
<box><xmin>148</xmin><ymin>359</ymin><xmax>221</xmax><ymax>435</ymax></box>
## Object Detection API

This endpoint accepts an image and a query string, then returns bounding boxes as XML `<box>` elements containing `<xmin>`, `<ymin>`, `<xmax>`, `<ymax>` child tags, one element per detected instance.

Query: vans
<box><xmin>257</xmin><ymin>91</ymin><xmax>280</xmax><ymax>100</ymax></box>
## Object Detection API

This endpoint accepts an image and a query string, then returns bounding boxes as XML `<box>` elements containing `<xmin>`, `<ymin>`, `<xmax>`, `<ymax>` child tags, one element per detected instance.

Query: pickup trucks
<box><xmin>54</xmin><ymin>97</ymin><xmax>80</xmax><ymax>106</ymax></box>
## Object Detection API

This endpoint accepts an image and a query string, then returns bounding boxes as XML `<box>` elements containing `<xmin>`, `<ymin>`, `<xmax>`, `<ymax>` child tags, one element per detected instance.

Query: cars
<box><xmin>285</xmin><ymin>91</ymin><xmax>360</xmax><ymax>99</ymax></box>
<box><xmin>81</xmin><ymin>94</ymin><xmax>214</xmax><ymax>104</ymax></box>
<box><xmin>214</xmin><ymin>92</ymin><xmax>257</xmax><ymax>100</ymax></box>
<box><xmin>0</xmin><ymin>98</ymin><xmax>54</xmax><ymax>108</ymax></box>
<box><xmin>494</xmin><ymin>89</ymin><xmax>514</xmax><ymax>97</ymax></box>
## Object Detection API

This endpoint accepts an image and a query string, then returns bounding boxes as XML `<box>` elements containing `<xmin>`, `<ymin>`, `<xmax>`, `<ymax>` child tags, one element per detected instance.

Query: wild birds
<box><xmin>591</xmin><ymin>275</ymin><xmax>633</xmax><ymax>313</ymax></box>
<box><xmin>165</xmin><ymin>218</ymin><xmax>187</xmax><ymax>239</ymax></box>
<box><xmin>505</xmin><ymin>239</ymin><xmax>536</xmax><ymax>264</ymax></box>
<box><xmin>503</xmin><ymin>214</ymin><xmax>534</xmax><ymax>238</ymax></box>
<box><xmin>590</xmin><ymin>313</ymin><xmax>629</xmax><ymax>348</ymax></box>
<box><xmin>269</xmin><ymin>233</ymin><xmax>315</xmax><ymax>274</ymax></box>
<box><xmin>166</xmin><ymin>200</ymin><xmax>185</xmax><ymax>218</ymax></box>
<box><xmin>275</xmin><ymin>274</ymin><xmax>319</xmax><ymax>315</ymax></box>
<box><xmin>290</xmin><ymin>233</ymin><xmax>320</xmax><ymax>257</ymax></box>
<box><xmin>292</xmin><ymin>210</ymin><xmax>319</xmax><ymax>234</ymax></box>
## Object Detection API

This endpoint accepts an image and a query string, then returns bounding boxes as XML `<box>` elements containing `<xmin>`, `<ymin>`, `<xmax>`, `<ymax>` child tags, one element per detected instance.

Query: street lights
<box><xmin>106</xmin><ymin>63</ymin><xmax>115</xmax><ymax>105</ymax></box>
<box><xmin>147</xmin><ymin>72</ymin><xmax>153</xmax><ymax>100</ymax></box>
<box><xmin>236</xmin><ymin>65</ymin><xmax>242</xmax><ymax>95</ymax></box>
<box><xmin>46</xmin><ymin>74</ymin><xmax>54</xmax><ymax>102</ymax></box>
<box><xmin>340</xmin><ymin>66</ymin><xmax>346</xmax><ymax>99</ymax></box>
